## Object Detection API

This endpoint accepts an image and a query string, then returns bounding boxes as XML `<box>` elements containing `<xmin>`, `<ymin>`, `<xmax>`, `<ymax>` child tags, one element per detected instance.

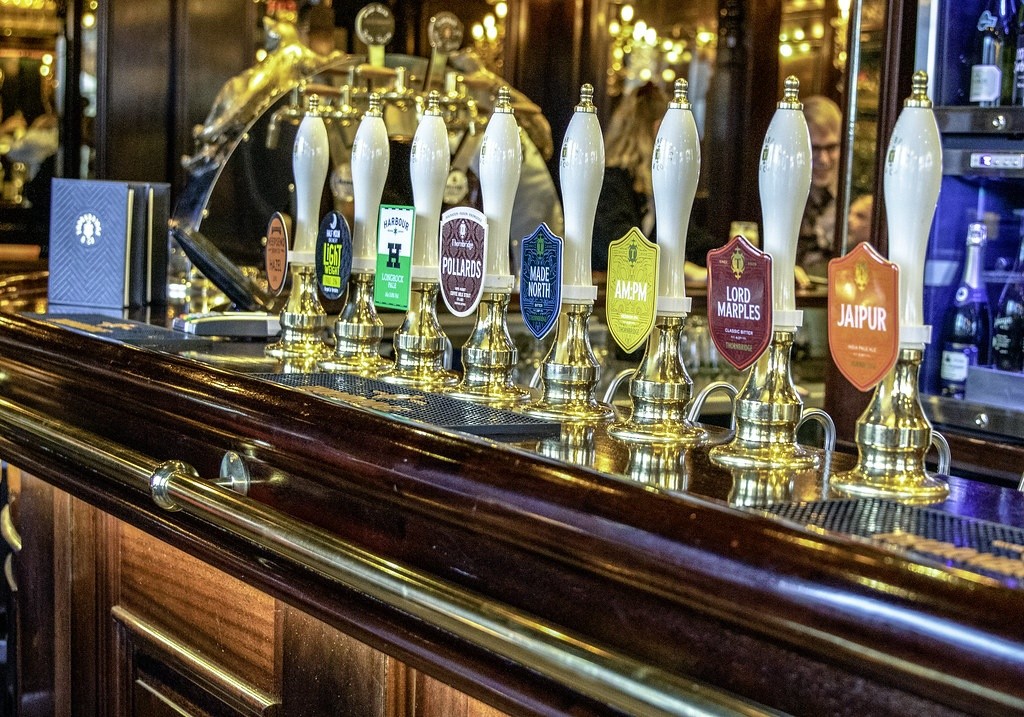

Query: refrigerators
<box><xmin>828</xmin><ymin>0</ymin><xmax>1024</xmax><ymax>490</ymax></box>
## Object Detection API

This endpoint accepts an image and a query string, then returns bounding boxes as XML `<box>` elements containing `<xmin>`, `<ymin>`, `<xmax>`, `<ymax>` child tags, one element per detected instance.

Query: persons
<box><xmin>3</xmin><ymin>95</ymin><xmax>57</xmax><ymax>258</ymax></box>
<box><xmin>800</xmin><ymin>95</ymin><xmax>876</xmax><ymax>271</ymax></box>
<box><xmin>582</xmin><ymin>82</ymin><xmax>708</xmax><ymax>286</ymax></box>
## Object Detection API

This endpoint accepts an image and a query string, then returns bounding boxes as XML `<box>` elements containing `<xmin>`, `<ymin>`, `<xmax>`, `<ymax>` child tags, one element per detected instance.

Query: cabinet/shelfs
<box><xmin>865</xmin><ymin>0</ymin><xmax>1024</xmax><ymax>475</ymax></box>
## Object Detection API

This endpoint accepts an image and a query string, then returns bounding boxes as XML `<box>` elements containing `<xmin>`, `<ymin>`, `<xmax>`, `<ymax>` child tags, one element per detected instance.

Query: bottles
<box><xmin>990</xmin><ymin>237</ymin><xmax>1024</xmax><ymax>372</ymax></box>
<box><xmin>936</xmin><ymin>224</ymin><xmax>990</xmax><ymax>400</ymax></box>
<box><xmin>967</xmin><ymin>0</ymin><xmax>1024</xmax><ymax>106</ymax></box>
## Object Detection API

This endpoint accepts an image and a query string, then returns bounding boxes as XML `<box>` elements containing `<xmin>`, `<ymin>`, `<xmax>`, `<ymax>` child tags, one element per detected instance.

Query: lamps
<box><xmin>264</xmin><ymin>92</ymin><xmax>334</xmax><ymax>359</ymax></box>
<box><xmin>607</xmin><ymin>76</ymin><xmax>738</xmax><ymax>443</ymax></box>
<box><xmin>446</xmin><ymin>85</ymin><xmax>531</xmax><ymax>401</ymax></box>
<box><xmin>520</xmin><ymin>83</ymin><xmax>638</xmax><ymax>428</ymax></box>
<box><xmin>709</xmin><ymin>74</ymin><xmax>834</xmax><ymax>476</ymax></box>
<box><xmin>318</xmin><ymin>90</ymin><xmax>394</xmax><ymax>374</ymax></box>
<box><xmin>828</xmin><ymin>70</ymin><xmax>950</xmax><ymax>507</ymax></box>
<box><xmin>376</xmin><ymin>89</ymin><xmax>461</xmax><ymax>389</ymax></box>
